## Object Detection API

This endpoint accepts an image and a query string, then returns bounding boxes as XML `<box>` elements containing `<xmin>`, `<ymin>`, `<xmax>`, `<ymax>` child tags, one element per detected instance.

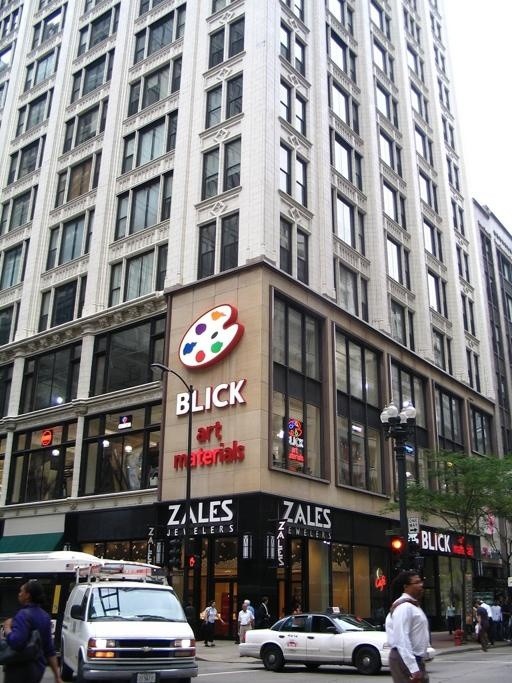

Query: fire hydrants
<box><xmin>454</xmin><ymin>627</ymin><xmax>465</xmax><ymax>645</ymax></box>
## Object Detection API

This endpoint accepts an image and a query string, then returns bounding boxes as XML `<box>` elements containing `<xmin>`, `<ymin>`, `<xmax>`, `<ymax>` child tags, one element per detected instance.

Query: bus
<box><xmin>0</xmin><ymin>545</ymin><xmax>164</xmax><ymax>655</ymax></box>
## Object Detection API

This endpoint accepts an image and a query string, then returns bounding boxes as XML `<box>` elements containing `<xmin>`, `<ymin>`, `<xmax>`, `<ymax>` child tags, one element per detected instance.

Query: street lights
<box><xmin>377</xmin><ymin>397</ymin><xmax>419</xmax><ymax>572</ymax></box>
<box><xmin>150</xmin><ymin>359</ymin><xmax>195</xmax><ymax>612</ymax></box>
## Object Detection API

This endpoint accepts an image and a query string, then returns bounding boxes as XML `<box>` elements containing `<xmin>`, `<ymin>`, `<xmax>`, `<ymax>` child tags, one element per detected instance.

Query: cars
<box><xmin>235</xmin><ymin>609</ymin><xmax>438</xmax><ymax>675</ymax></box>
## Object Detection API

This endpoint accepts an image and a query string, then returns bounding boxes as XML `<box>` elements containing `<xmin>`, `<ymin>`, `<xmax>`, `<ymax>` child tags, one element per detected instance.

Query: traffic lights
<box><xmin>389</xmin><ymin>537</ymin><xmax>405</xmax><ymax>552</ymax></box>
<box><xmin>188</xmin><ymin>554</ymin><xmax>201</xmax><ymax>568</ymax></box>
<box><xmin>167</xmin><ymin>536</ymin><xmax>183</xmax><ymax>568</ymax></box>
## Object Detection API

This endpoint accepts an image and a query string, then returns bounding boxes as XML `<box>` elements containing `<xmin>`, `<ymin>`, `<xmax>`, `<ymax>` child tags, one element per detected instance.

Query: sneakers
<box><xmin>205</xmin><ymin>644</ymin><xmax>215</xmax><ymax>648</ymax></box>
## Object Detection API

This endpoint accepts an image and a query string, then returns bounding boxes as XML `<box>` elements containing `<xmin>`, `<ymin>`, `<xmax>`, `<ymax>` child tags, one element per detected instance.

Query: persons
<box><xmin>293</xmin><ymin>602</ymin><xmax>302</xmax><ymax>615</ymax></box>
<box><xmin>0</xmin><ymin>582</ymin><xmax>62</xmax><ymax>683</ymax></box>
<box><xmin>237</xmin><ymin>596</ymin><xmax>273</xmax><ymax>645</ymax></box>
<box><xmin>383</xmin><ymin>571</ymin><xmax>434</xmax><ymax>683</ymax></box>
<box><xmin>445</xmin><ymin>600</ymin><xmax>507</xmax><ymax>651</ymax></box>
<box><xmin>204</xmin><ymin>601</ymin><xmax>226</xmax><ymax>647</ymax></box>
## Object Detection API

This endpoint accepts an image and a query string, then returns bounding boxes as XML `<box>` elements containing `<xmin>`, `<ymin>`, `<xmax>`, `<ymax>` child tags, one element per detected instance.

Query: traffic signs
<box><xmin>409</xmin><ymin>516</ymin><xmax>421</xmax><ymax>536</ymax></box>
<box><xmin>382</xmin><ymin>526</ymin><xmax>403</xmax><ymax>535</ymax></box>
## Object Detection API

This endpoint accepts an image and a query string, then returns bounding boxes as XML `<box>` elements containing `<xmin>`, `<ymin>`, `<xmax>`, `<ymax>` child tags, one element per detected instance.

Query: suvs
<box><xmin>55</xmin><ymin>555</ymin><xmax>202</xmax><ymax>682</ymax></box>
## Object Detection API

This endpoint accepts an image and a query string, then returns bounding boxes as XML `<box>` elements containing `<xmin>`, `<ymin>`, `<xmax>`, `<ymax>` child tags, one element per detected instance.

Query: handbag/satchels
<box><xmin>0</xmin><ymin>630</ymin><xmax>44</xmax><ymax>665</ymax></box>
<box><xmin>200</xmin><ymin>611</ymin><xmax>208</xmax><ymax>620</ymax></box>
